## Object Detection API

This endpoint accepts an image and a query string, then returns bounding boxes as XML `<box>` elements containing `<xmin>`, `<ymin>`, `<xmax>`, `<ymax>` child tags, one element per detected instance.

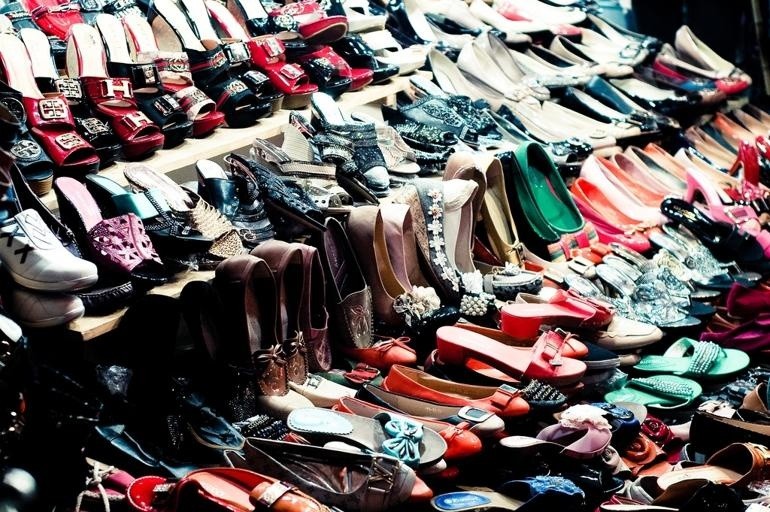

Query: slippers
<box><xmin>0</xmin><ymin>0</ymin><xmax>770</xmax><ymax>511</ymax></box>
